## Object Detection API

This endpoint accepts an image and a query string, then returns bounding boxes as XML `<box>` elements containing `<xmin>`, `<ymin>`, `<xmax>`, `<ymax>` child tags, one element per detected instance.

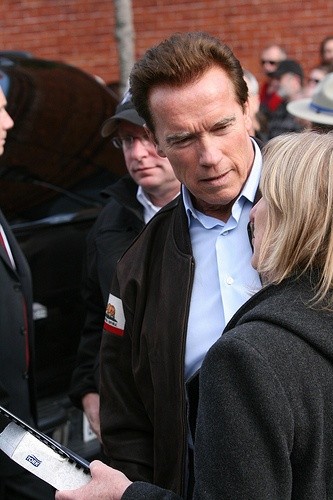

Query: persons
<box><xmin>97</xmin><ymin>32</ymin><xmax>272</xmax><ymax>500</ymax></box>
<box><xmin>257</xmin><ymin>40</ymin><xmax>333</xmax><ymax>143</ymax></box>
<box><xmin>0</xmin><ymin>76</ymin><xmax>40</xmax><ymax>500</ymax></box>
<box><xmin>68</xmin><ymin>93</ymin><xmax>177</xmax><ymax>446</ymax></box>
<box><xmin>54</xmin><ymin>129</ymin><xmax>333</xmax><ymax>500</ymax></box>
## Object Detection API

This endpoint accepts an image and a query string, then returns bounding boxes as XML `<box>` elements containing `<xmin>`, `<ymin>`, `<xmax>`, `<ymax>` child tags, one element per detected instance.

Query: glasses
<box><xmin>109</xmin><ymin>133</ymin><xmax>152</xmax><ymax>149</ymax></box>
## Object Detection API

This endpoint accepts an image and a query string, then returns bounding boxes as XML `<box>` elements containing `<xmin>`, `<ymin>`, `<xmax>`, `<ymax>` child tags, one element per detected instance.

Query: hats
<box><xmin>101</xmin><ymin>88</ymin><xmax>148</xmax><ymax>138</ymax></box>
<box><xmin>285</xmin><ymin>72</ymin><xmax>333</xmax><ymax>125</ymax></box>
<box><xmin>266</xmin><ymin>60</ymin><xmax>303</xmax><ymax>80</ymax></box>
